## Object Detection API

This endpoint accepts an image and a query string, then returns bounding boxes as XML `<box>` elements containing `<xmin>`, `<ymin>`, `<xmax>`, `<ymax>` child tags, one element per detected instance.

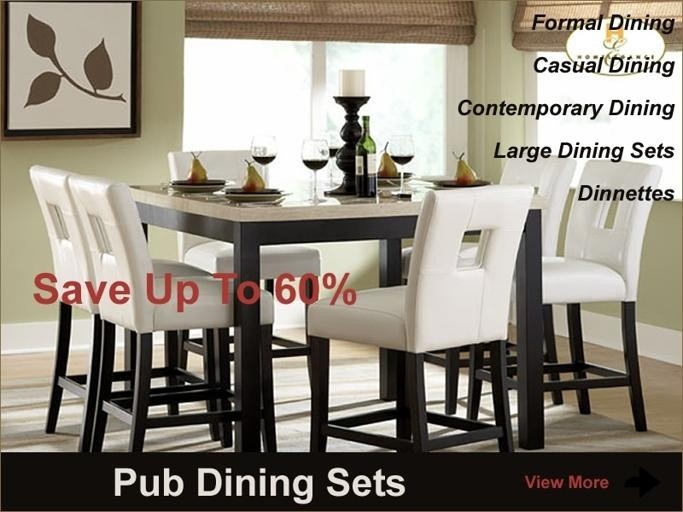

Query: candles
<box><xmin>340</xmin><ymin>69</ymin><xmax>365</xmax><ymax>97</ymax></box>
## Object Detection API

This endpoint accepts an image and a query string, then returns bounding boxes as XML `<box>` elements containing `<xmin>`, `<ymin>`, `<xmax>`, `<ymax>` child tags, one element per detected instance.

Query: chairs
<box><xmin>26</xmin><ymin>148</ymin><xmax>663</xmax><ymax>452</ymax></box>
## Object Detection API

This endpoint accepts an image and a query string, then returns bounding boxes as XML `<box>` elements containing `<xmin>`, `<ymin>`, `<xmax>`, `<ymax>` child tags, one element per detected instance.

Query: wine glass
<box><xmin>387</xmin><ymin>135</ymin><xmax>421</xmax><ymax>194</ymax></box>
<box><xmin>304</xmin><ymin>130</ymin><xmax>343</xmax><ymax>205</ymax></box>
<box><xmin>251</xmin><ymin>136</ymin><xmax>275</xmax><ymax>189</ymax></box>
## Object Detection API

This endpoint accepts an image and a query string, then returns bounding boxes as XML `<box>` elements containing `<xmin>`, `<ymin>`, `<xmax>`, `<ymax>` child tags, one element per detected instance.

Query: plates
<box><xmin>170</xmin><ymin>179</ymin><xmax>288</xmax><ymax>203</ymax></box>
<box><xmin>374</xmin><ymin>171</ymin><xmax>414</xmax><ymax>188</ymax></box>
<box><xmin>420</xmin><ymin>174</ymin><xmax>490</xmax><ymax>190</ymax></box>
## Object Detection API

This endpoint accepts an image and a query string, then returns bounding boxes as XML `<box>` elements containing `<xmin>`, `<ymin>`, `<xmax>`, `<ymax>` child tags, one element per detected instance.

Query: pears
<box><xmin>452</xmin><ymin>151</ymin><xmax>477</xmax><ymax>185</ymax></box>
<box><xmin>186</xmin><ymin>151</ymin><xmax>206</xmax><ymax>183</ymax></box>
<box><xmin>377</xmin><ymin>141</ymin><xmax>398</xmax><ymax>178</ymax></box>
<box><xmin>240</xmin><ymin>159</ymin><xmax>265</xmax><ymax>193</ymax></box>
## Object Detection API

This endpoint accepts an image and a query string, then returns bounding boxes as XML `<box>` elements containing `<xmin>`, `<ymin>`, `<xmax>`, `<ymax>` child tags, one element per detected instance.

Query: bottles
<box><xmin>354</xmin><ymin>116</ymin><xmax>378</xmax><ymax>198</ymax></box>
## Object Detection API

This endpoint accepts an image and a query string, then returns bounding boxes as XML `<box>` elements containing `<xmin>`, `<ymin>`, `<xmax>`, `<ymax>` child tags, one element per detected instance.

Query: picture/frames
<box><xmin>0</xmin><ymin>0</ymin><xmax>143</xmax><ymax>144</ymax></box>
